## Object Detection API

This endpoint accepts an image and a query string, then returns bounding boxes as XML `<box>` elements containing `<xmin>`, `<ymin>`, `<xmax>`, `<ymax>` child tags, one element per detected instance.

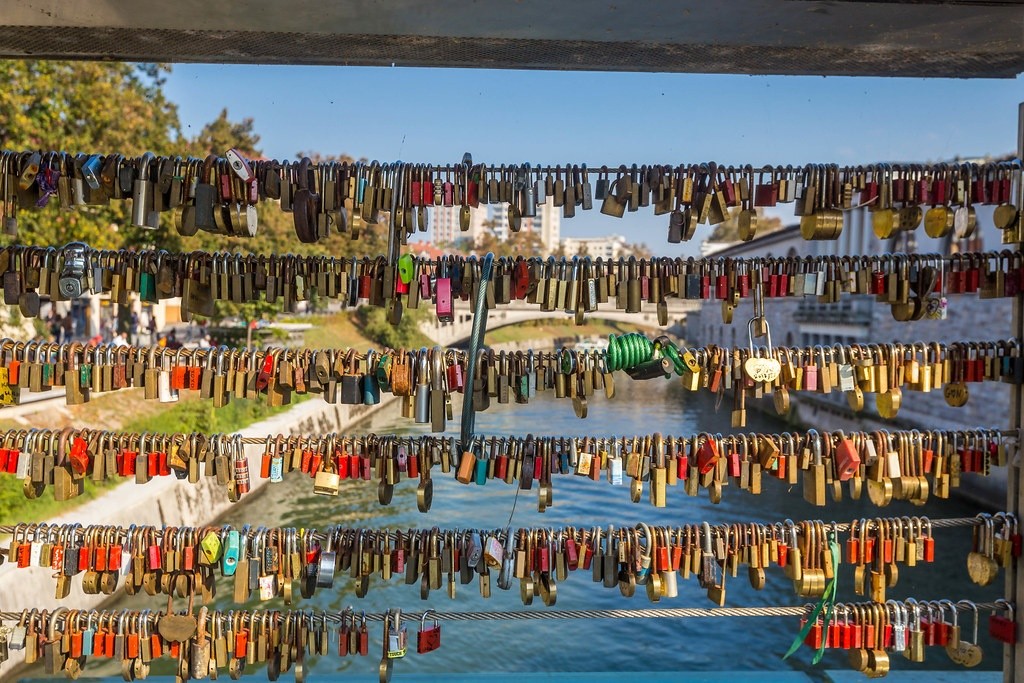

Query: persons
<box><xmin>45</xmin><ymin>309</ymin><xmax>277</xmax><ymax>350</ymax></box>
